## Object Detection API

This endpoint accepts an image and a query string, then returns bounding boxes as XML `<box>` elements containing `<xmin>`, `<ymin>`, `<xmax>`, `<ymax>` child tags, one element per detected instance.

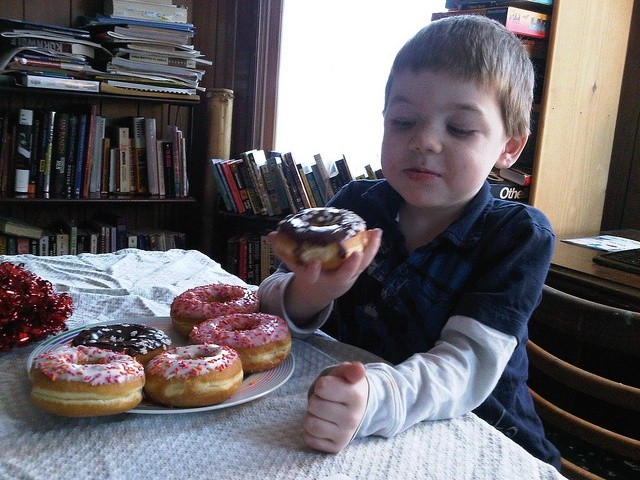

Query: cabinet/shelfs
<box><xmin>1</xmin><ymin>72</ymin><xmax>232</xmax><ymax>289</ymax></box>
<box><xmin>448</xmin><ymin>228</ymin><xmax>453</xmax><ymax>238</ymax></box>
<box><xmin>444</xmin><ymin>0</ymin><xmax>632</xmax><ymax>232</ymax></box>
<box><xmin>215</xmin><ymin>203</ymin><xmax>285</xmax><ymax>287</ymax></box>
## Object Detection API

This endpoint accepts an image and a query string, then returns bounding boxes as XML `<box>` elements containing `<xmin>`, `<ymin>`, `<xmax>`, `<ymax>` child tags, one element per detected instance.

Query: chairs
<box><xmin>527</xmin><ymin>279</ymin><xmax>640</xmax><ymax>478</ymax></box>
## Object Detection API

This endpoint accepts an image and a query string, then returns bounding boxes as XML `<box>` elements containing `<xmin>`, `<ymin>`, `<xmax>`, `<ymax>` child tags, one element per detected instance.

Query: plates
<box><xmin>25</xmin><ymin>316</ymin><xmax>296</xmax><ymax>415</ymax></box>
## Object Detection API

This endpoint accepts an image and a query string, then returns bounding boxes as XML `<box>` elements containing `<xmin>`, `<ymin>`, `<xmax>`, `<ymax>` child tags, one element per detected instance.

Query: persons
<box><xmin>257</xmin><ymin>14</ymin><xmax>565</xmax><ymax>477</ymax></box>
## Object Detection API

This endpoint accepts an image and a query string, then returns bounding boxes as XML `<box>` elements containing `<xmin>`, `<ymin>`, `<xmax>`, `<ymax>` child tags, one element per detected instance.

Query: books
<box><xmin>211</xmin><ymin>149</ymin><xmax>384</xmax><ymax>217</ymax></box>
<box><xmin>1</xmin><ymin>210</ymin><xmax>187</xmax><ymax>256</ymax></box>
<box><xmin>227</xmin><ymin>225</ymin><xmax>279</xmax><ymax>286</ymax></box>
<box><xmin>498</xmin><ymin>168</ymin><xmax>532</xmax><ymax>186</ymax></box>
<box><xmin>1</xmin><ymin>103</ymin><xmax>191</xmax><ymax>199</ymax></box>
<box><xmin>0</xmin><ymin>0</ymin><xmax>214</xmax><ymax>101</ymax></box>
<box><xmin>559</xmin><ymin>233</ymin><xmax>639</xmax><ymax>252</ymax></box>
<box><xmin>428</xmin><ymin>0</ymin><xmax>554</xmax><ymax>105</ymax></box>
<box><xmin>486</xmin><ymin>170</ymin><xmax>530</xmax><ymax>200</ymax></box>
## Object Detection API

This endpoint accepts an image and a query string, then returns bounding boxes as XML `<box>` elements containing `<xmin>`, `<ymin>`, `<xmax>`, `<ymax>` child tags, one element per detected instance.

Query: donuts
<box><xmin>29</xmin><ymin>349</ymin><xmax>146</xmax><ymax>418</ymax></box>
<box><xmin>169</xmin><ymin>283</ymin><xmax>260</xmax><ymax>336</ymax></box>
<box><xmin>71</xmin><ymin>322</ymin><xmax>173</xmax><ymax>366</ymax></box>
<box><xmin>189</xmin><ymin>313</ymin><xmax>293</xmax><ymax>374</ymax></box>
<box><xmin>142</xmin><ymin>344</ymin><xmax>245</xmax><ymax>408</ymax></box>
<box><xmin>274</xmin><ymin>205</ymin><xmax>370</xmax><ymax>271</ymax></box>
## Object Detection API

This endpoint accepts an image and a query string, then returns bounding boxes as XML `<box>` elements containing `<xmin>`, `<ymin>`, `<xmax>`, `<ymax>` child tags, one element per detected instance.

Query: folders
<box><xmin>0</xmin><ymin>17</ymin><xmax>91</xmax><ymax>38</ymax></box>
<box><xmin>79</xmin><ymin>16</ymin><xmax>197</xmax><ymax>33</ymax></box>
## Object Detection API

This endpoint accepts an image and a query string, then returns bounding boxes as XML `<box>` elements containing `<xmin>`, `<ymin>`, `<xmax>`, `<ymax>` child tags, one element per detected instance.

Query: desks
<box><xmin>519</xmin><ymin>229</ymin><xmax>640</xmax><ymax>476</ymax></box>
